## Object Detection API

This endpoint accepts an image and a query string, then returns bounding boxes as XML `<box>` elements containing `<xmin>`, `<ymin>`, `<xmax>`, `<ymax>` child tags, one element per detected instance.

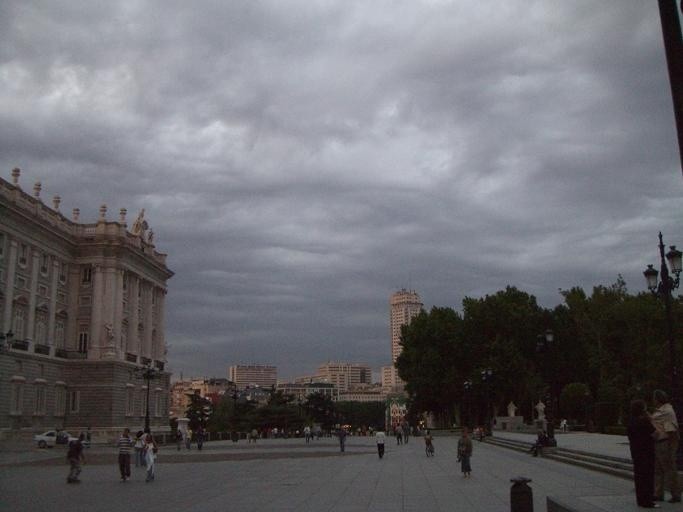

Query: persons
<box><xmin>331</xmin><ymin>424</ymin><xmax>337</xmax><ymax>440</ymax></box>
<box><xmin>133</xmin><ymin>431</ymin><xmax>146</xmax><ymax>469</ymax></box>
<box><xmin>143</xmin><ymin>428</ymin><xmax>154</xmax><ymax>465</ymax></box>
<box><xmin>262</xmin><ymin>425</ymin><xmax>278</xmax><ymax>439</ymax></box>
<box><xmin>477</xmin><ymin>425</ymin><xmax>492</xmax><ymax>442</ymax></box>
<box><xmin>104</xmin><ymin>322</ymin><xmax>115</xmax><ymax>343</ymax></box>
<box><xmin>646</xmin><ymin>389</ymin><xmax>682</xmax><ymax>502</ymax></box>
<box><xmin>424</xmin><ymin>431</ymin><xmax>434</xmax><ymax>457</ymax></box>
<box><xmin>143</xmin><ymin>441</ymin><xmax>158</xmax><ymax>482</ymax></box>
<box><xmin>387</xmin><ymin>423</ymin><xmax>409</xmax><ymax>444</ymax></box>
<box><xmin>337</xmin><ymin>428</ymin><xmax>346</xmax><ymax>451</ymax></box>
<box><xmin>3</xmin><ymin>329</ymin><xmax>14</xmax><ymax>349</ymax></box>
<box><xmin>369</xmin><ymin>425</ymin><xmax>374</xmax><ymax>436</ymax></box>
<box><xmin>412</xmin><ymin>426</ymin><xmax>418</xmax><ymax>436</ymax></box>
<box><xmin>85</xmin><ymin>426</ymin><xmax>92</xmax><ymax>447</ymax></box>
<box><xmin>376</xmin><ymin>428</ymin><xmax>385</xmax><ymax>458</ymax></box>
<box><xmin>176</xmin><ymin>428</ymin><xmax>206</xmax><ymax>450</ymax></box>
<box><xmin>457</xmin><ymin>432</ymin><xmax>473</xmax><ymax>477</ymax></box>
<box><xmin>303</xmin><ymin>424</ymin><xmax>322</xmax><ymax>444</ymax></box>
<box><xmin>231</xmin><ymin>426</ymin><xmax>262</xmax><ymax>444</ymax></box>
<box><xmin>116</xmin><ymin>428</ymin><xmax>135</xmax><ymax>481</ymax></box>
<box><xmin>529</xmin><ymin>427</ymin><xmax>556</xmax><ymax>457</ymax></box>
<box><xmin>64</xmin><ymin>434</ymin><xmax>86</xmax><ymax>484</ymax></box>
<box><xmin>623</xmin><ymin>399</ymin><xmax>662</xmax><ymax>509</ymax></box>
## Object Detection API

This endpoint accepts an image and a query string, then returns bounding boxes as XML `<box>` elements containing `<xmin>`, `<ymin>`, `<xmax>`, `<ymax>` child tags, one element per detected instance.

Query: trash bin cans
<box><xmin>510</xmin><ymin>477</ymin><xmax>533</xmax><ymax>512</ymax></box>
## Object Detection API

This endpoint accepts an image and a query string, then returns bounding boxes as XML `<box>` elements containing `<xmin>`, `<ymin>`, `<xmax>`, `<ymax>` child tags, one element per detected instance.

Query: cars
<box><xmin>32</xmin><ymin>430</ymin><xmax>90</xmax><ymax>449</ymax></box>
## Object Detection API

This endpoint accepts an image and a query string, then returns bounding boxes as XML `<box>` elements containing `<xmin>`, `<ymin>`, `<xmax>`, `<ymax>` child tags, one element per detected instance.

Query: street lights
<box><xmin>480</xmin><ymin>367</ymin><xmax>493</xmax><ymax>416</ymax></box>
<box><xmin>534</xmin><ymin>328</ymin><xmax>560</xmax><ymax>447</ymax></box>
<box><xmin>230</xmin><ymin>385</ymin><xmax>239</xmax><ymax>411</ymax></box>
<box><xmin>644</xmin><ymin>232</ymin><xmax>681</xmax><ymax>402</ymax></box>
<box><xmin>142</xmin><ymin>359</ymin><xmax>159</xmax><ymax>434</ymax></box>
<box><xmin>464</xmin><ymin>378</ymin><xmax>474</xmax><ymax>428</ymax></box>
<box><xmin>0</xmin><ymin>330</ymin><xmax>12</xmax><ymax>353</ymax></box>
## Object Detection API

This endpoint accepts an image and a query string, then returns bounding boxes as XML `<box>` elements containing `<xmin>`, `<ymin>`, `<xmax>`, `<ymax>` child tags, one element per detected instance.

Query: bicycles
<box><xmin>424</xmin><ymin>444</ymin><xmax>434</xmax><ymax>457</ymax></box>
